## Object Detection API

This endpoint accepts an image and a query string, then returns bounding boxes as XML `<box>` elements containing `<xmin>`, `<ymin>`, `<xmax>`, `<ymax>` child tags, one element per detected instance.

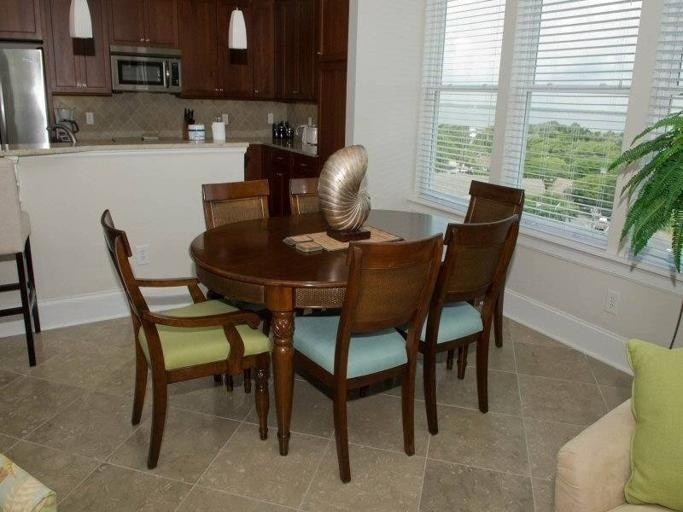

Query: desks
<box><xmin>190</xmin><ymin>210</ymin><xmax>464</xmax><ymax>455</ymax></box>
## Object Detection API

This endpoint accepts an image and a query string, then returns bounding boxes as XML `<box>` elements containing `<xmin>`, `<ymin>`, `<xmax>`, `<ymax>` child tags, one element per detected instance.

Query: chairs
<box><xmin>438</xmin><ymin>181</ymin><xmax>524</xmax><ymax>369</ymax></box>
<box><xmin>291</xmin><ymin>177</ymin><xmax>319</xmax><ymax>316</ymax></box>
<box><xmin>393</xmin><ymin>215</ymin><xmax>520</xmax><ymax>435</ymax></box>
<box><xmin>100</xmin><ymin>210</ymin><xmax>270</xmax><ymax>469</ymax></box>
<box><xmin>201</xmin><ymin>179</ymin><xmax>305</xmax><ymax>338</ymax></box>
<box><xmin>294</xmin><ymin>232</ymin><xmax>443</xmax><ymax>486</ymax></box>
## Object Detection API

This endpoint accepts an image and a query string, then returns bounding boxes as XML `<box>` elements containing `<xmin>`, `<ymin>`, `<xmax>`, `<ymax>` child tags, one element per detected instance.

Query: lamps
<box><xmin>69</xmin><ymin>0</ymin><xmax>92</xmax><ymax>39</ymax></box>
<box><xmin>228</xmin><ymin>0</ymin><xmax>247</xmax><ymax>49</ymax></box>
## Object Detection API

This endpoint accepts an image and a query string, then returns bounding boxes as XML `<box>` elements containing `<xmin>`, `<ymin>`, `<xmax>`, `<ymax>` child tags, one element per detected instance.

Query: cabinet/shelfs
<box><xmin>107</xmin><ymin>0</ymin><xmax>180</xmax><ymax>48</ymax></box>
<box><xmin>39</xmin><ymin>1</ymin><xmax>111</xmax><ymax>95</ymax></box>
<box><xmin>317</xmin><ymin>1</ymin><xmax>350</xmax><ymax>157</ymax></box>
<box><xmin>275</xmin><ymin>0</ymin><xmax>320</xmax><ymax>103</ymax></box>
<box><xmin>262</xmin><ymin>145</ymin><xmax>319</xmax><ymax>218</ymax></box>
<box><xmin>178</xmin><ymin>0</ymin><xmax>277</xmax><ymax>103</ymax></box>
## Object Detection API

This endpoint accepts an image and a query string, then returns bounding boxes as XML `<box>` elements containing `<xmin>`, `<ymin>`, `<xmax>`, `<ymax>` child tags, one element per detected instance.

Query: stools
<box><xmin>0</xmin><ymin>235</ymin><xmax>42</xmax><ymax>368</ymax></box>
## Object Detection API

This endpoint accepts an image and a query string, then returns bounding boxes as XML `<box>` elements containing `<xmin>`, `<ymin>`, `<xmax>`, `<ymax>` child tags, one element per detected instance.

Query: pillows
<box><xmin>625</xmin><ymin>340</ymin><xmax>683</xmax><ymax>512</ymax></box>
